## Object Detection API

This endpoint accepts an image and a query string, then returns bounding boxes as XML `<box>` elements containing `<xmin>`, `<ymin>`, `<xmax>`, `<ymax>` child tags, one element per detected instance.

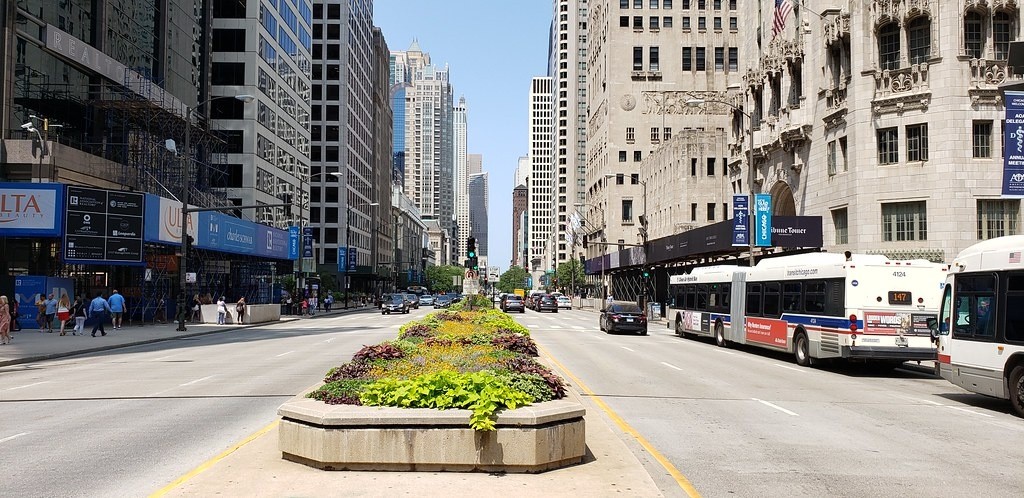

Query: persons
<box><xmin>324</xmin><ymin>294</ymin><xmax>333</xmax><ymax>313</ymax></box>
<box><xmin>978</xmin><ymin>302</ymin><xmax>988</xmax><ymax>315</ymax></box>
<box><xmin>352</xmin><ymin>292</ymin><xmax>366</xmax><ymax>309</ymax></box>
<box><xmin>155</xmin><ymin>293</ymin><xmax>165</xmax><ymax>324</ymax></box>
<box><xmin>56</xmin><ymin>292</ymin><xmax>70</xmax><ymax>336</ymax></box>
<box><xmin>191</xmin><ymin>292</ymin><xmax>213</xmax><ymax>323</ymax></box>
<box><xmin>0</xmin><ymin>296</ymin><xmax>12</xmax><ymax>345</ymax></box>
<box><xmin>287</xmin><ymin>295</ymin><xmax>292</xmax><ymax>315</ymax></box>
<box><xmin>236</xmin><ymin>298</ymin><xmax>246</xmax><ymax>325</ymax></box>
<box><xmin>217</xmin><ymin>297</ymin><xmax>227</xmax><ymax>325</ymax></box>
<box><xmin>72</xmin><ymin>296</ymin><xmax>88</xmax><ymax>336</ymax></box>
<box><xmin>35</xmin><ymin>293</ymin><xmax>58</xmax><ymax>333</ymax></box>
<box><xmin>300</xmin><ymin>295</ymin><xmax>315</xmax><ymax>316</ymax></box>
<box><xmin>108</xmin><ymin>290</ymin><xmax>127</xmax><ymax>329</ymax></box>
<box><xmin>575</xmin><ymin>288</ymin><xmax>586</xmax><ymax>299</ymax></box>
<box><xmin>89</xmin><ymin>291</ymin><xmax>113</xmax><ymax>337</ymax></box>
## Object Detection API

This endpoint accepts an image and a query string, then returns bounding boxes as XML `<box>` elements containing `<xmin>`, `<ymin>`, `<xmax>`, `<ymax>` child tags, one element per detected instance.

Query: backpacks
<box><xmin>236</xmin><ymin>301</ymin><xmax>244</xmax><ymax>311</ymax></box>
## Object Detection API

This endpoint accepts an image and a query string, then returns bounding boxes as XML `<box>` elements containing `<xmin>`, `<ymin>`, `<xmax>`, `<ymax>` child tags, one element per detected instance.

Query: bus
<box><xmin>667</xmin><ymin>252</ymin><xmax>950</xmax><ymax>367</ymax></box>
<box><xmin>926</xmin><ymin>235</ymin><xmax>1024</xmax><ymax>418</ymax></box>
<box><xmin>407</xmin><ymin>286</ymin><xmax>428</xmax><ymax>298</ymax></box>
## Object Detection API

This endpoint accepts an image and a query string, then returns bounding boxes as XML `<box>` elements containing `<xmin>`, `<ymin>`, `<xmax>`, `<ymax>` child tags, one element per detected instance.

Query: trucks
<box><xmin>514</xmin><ymin>289</ymin><xmax>524</xmax><ymax>298</ymax></box>
<box><xmin>526</xmin><ymin>290</ymin><xmax>546</xmax><ymax>308</ymax></box>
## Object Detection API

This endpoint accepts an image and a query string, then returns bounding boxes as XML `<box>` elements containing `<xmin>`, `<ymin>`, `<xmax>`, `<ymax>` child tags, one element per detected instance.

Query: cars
<box><xmin>434</xmin><ymin>293</ymin><xmax>462</xmax><ymax>309</ymax></box>
<box><xmin>418</xmin><ymin>295</ymin><xmax>433</xmax><ymax>305</ymax></box>
<box><xmin>551</xmin><ymin>293</ymin><xmax>572</xmax><ymax>310</ymax></box>
<box><xmin>407</xmin><ymin>294</ymin><xmax>419</xmax><ymax>309</ymax></box>
<box><xmin>599</xmin><ymin>303</ymin><xmax>647</xmax><ymax>336</ymax></box>
<box><xmin>381</xmin><ymin>294</ymin><xmax>409</xmax><ymax>314</ymax></box>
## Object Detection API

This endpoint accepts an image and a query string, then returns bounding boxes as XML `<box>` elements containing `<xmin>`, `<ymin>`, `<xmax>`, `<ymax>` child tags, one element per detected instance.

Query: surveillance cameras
<box><xmin>21</xmin><ymin>122</ymin><xmax>33</xmax><ymax>129</ymax></box>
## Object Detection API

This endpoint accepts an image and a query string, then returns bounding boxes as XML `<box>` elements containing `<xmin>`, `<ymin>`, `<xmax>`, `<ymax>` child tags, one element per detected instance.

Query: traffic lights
<box><xmin>467</xmin><ymin>237</ymin><xmax>476</xmax><ymax>258</ymax></box>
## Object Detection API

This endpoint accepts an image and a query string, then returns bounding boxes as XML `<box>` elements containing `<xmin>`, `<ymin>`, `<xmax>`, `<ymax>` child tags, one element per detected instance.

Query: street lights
<box><xmin>685</xmin><ymin>99</ymin><xmax>755</xmax><ymax>267</ymax></box>
<box><xmin>344</xmin><ymin>203</ymin><xmax>380</xmax><ymax>308</ymax></box>
<box><xmin>178</xmin><ymin>95</ymin><xmax>255</xmax><ymax>330</ymax></box>
<box><xmin>605</xmin><ymin>173</ymin><xmax>647</xmax><ymax>315</ymax></box>
<box><xmin>299</xmin><ymin>173</ymin><xmax>344</xmax><ymax>315</ymax></box>
<box><xmin>573</xmin><ymin>203</ymin><xmax>606</xmax><ymax>313</ymax></box>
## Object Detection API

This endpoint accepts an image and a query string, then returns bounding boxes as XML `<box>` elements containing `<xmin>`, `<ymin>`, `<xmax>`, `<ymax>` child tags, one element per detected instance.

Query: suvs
<box><xmin>530</xmin><ymin>293</ymin><xmax>557</xmax><ymax>312</ymax></box>
<box><xmin>494</xmin><ymin>293</ymin><xmax>524</xmax><ymax>313</ymax></box>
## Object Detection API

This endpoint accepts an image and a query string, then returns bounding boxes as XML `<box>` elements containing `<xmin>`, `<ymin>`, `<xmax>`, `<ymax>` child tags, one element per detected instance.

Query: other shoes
<box><xmin>117</xmin><ymin>327</ymin><xmax>121</xmax><ymax>329</ymax></box>
<box><xmin>38</xmin><ymin>326</ymin><xmax>45</xmax><ymax>332</ymax></box>
<box><xmin>91</xmin><ymin>333</ymin><xmax>96</xmax><ymax>337</ymax></box>
<box><xmin>0</xmin><ymin>340</ymin><xmax>7</xmax><ymax>345</ymax></box>
<box><xmin>102</xmin><ymin>333</ymin><xmax>107</xmax><ymax>336</ymax></box>
<box><xmin>113</xmin><ymin>326</ymin><xmax>116</xmax><ymax>328</ymax></box>
<box><xmin>47</xmin><ymin>329</ymin><xmax>52</xmax><ymax>333</ymax></box>
<box><xmin>73</xmin><ymin>330</ymin><xmax>75</xmax><ymax>335</ymax></box>
<box><xmin>8</xmin><ymin>335</ymin><xmax>13</xmax><ymax>343</ymax></box>
<box><xmin>60</xmin><ymin>332</ymin><xmax>65</xmax><ymax>336</ymax></box>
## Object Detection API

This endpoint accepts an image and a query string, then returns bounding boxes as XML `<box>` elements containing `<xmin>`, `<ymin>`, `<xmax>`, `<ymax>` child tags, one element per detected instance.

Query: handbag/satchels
<box><xmin>217</xmin><ymin>306</ymin><xmax>225</xmax><ymax>314</ymax></box>
